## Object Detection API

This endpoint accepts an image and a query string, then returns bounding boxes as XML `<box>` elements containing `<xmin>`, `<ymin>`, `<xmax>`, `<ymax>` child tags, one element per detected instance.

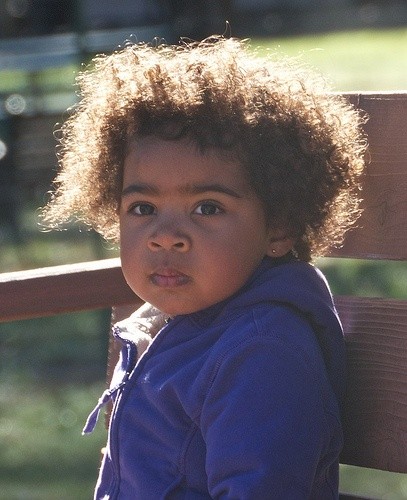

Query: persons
<box><xmin>35</xmin><ymin>34</ymin><xmax>370</xmax><ymax>500</ymax></box>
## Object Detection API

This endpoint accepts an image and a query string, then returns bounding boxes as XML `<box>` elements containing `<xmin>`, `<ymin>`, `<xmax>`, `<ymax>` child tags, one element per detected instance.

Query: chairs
<box><xmin>2</xmin><ymin>89</ymin><xmax>407</xmax><ymax>500</ymax></box>
